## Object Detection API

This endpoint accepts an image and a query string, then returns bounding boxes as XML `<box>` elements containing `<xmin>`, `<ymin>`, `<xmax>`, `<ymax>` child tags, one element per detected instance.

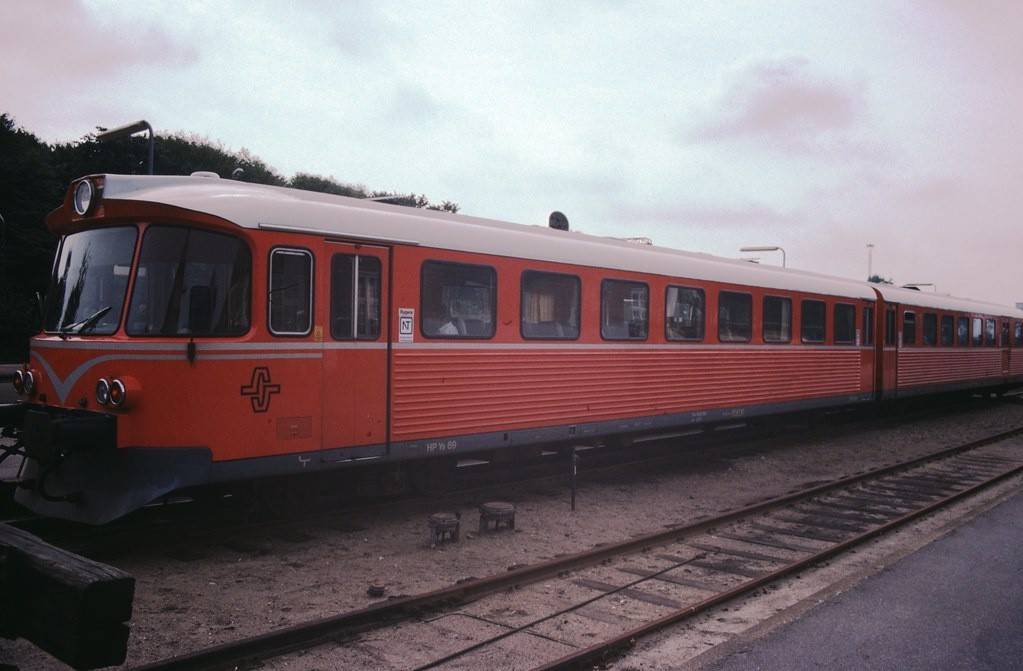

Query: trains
<box><xmin>1</xmin><ymin>166</ymin><xmax>1023</xmax><ymax>537</ymax></box>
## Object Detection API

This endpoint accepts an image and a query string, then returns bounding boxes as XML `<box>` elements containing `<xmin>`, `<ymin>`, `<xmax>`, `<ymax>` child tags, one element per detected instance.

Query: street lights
<box><xmin>737</xmin><ymin>247</ymin><xmax>787</xmax><ymax>269</ymax></box>
<box><xmin>906</xmin><ymin>282</ymin><xmax>937</xmax><ymax>293</ymax></box>
<box><xmin>96</xmin><ymin>118</ymin><xmax>158</xmax><ymax>178</ymax></box>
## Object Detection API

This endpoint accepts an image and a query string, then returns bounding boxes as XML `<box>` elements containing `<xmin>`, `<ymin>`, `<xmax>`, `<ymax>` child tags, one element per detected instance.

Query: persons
<box><xmin>423</xmin><ymin>305</ymin><xmax>459</xmax><ymax>335</ymax></box>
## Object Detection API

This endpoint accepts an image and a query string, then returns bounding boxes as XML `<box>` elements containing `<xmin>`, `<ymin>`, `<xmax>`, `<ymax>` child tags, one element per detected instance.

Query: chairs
<box><xmin>451</xmin><ymin>316</ymin><xmax>565</xmax><ymax>338</ymax></box>
